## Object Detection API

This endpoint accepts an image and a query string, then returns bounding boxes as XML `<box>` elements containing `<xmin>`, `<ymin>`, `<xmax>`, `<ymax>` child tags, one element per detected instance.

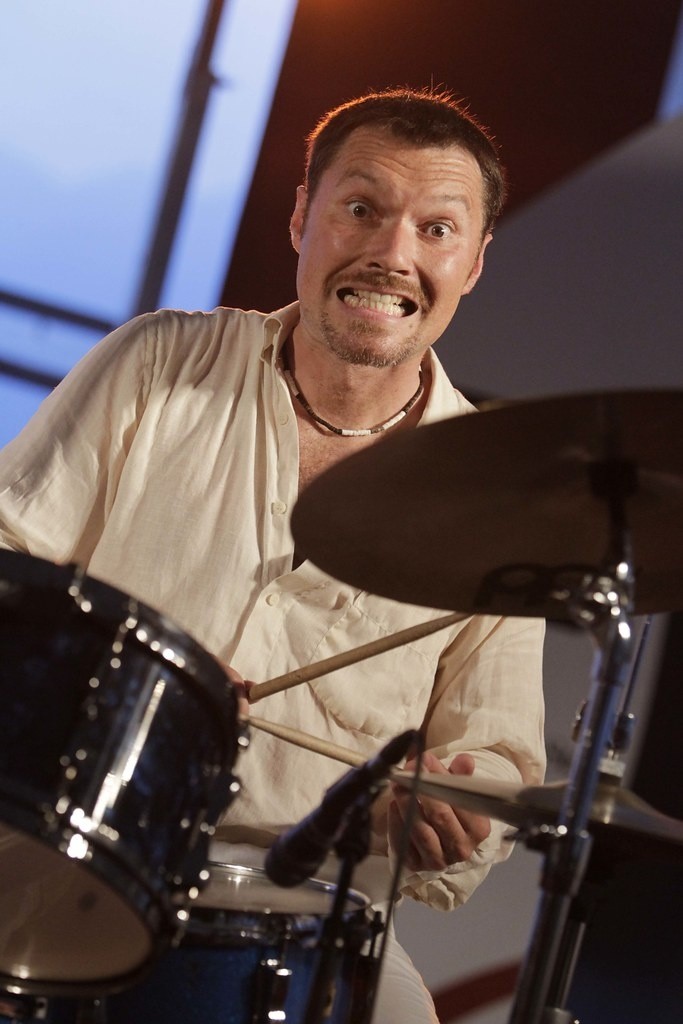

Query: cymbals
<box><xmin>287</xmin><ymin>387</ymin><xmax>683</xmax><ymax>627</ymax></box>
<box><xmin>392</xmin><ymin>772</ymin><xmax>683</xmax><ymax>850</ymax></box>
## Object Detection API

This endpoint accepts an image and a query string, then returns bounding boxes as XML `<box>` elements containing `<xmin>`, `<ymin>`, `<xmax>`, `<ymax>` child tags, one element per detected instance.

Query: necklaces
<box><xmin>282</xmin><ymin>343</ymin><xmax>423</xmax><ymax>437</ymax></box>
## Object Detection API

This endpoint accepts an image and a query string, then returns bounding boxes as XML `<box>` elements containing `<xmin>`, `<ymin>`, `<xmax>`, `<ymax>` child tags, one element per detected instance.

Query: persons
<box><xmin>0</xmin><ymin>93</ymin><xmax>546</xmax><ymax>1024</ymax></box>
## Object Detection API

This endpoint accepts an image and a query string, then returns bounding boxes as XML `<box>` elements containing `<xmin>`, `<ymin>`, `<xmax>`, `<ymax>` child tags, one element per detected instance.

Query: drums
<box><xmin>157</xmin><ymin>866</ymin><xmax>382</xmax><ymax>1024</ymax></box>
<box><xmin>0</xmin><ymin>551</ymin><xmax>251</xmax><ymax>1005</ymax></box>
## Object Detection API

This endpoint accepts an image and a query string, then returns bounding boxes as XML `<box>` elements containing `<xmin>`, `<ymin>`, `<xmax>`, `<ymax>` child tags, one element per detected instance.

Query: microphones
<box><xmin>264</xmin><ymin>730</ymin><xmax>416</xmax><ymax>888</ymax></box>
